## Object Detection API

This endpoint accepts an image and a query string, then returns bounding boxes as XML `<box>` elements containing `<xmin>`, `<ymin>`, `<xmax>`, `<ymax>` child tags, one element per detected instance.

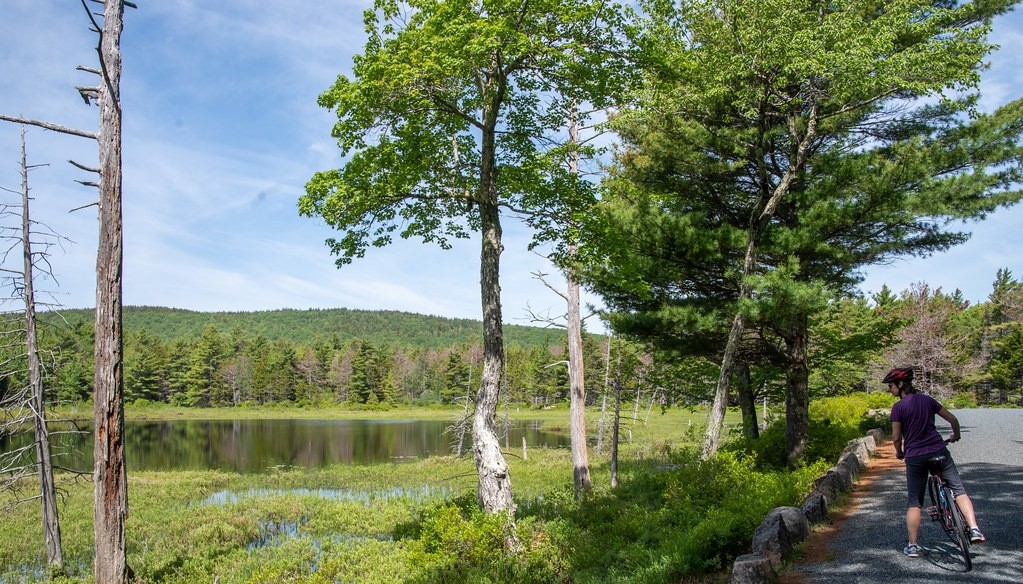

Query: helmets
<box><xmin>882</xmin><ymin>368</ymin><xmax>914</xmax><ymax>383</ymax></box>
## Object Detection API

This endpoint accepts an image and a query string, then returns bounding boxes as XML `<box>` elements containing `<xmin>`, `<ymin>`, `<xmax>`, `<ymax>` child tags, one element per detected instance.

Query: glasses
<box><xmin>889</xmin><ymin>384</ymin><xmax>899</xmax><ymax>389</ymax></box>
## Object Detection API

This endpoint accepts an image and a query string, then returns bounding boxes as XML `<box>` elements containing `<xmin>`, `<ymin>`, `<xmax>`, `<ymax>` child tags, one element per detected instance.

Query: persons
<box><xmin>882</xmin><ymin>368</ymin><xmax>985</xmax><ymax>557</ymax></box>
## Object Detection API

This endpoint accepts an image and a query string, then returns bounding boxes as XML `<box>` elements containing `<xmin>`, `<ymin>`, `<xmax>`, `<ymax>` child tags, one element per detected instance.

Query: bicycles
<box><xmin>896</xmin><ymin>437</ymin><xmax>973</xmax><ymax>571</ymax></box>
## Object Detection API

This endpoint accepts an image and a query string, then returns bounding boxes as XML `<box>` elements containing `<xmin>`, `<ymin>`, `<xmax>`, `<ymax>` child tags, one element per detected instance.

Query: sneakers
<box><xmin>969</xmin><ymin>527</ymin><xmax>986</xmax><ymax>542</ymax></box>
<box><xmin>903</xmin><ymin>543</ymin><xmax>920</xmax><ymax>556</ymax></box>
